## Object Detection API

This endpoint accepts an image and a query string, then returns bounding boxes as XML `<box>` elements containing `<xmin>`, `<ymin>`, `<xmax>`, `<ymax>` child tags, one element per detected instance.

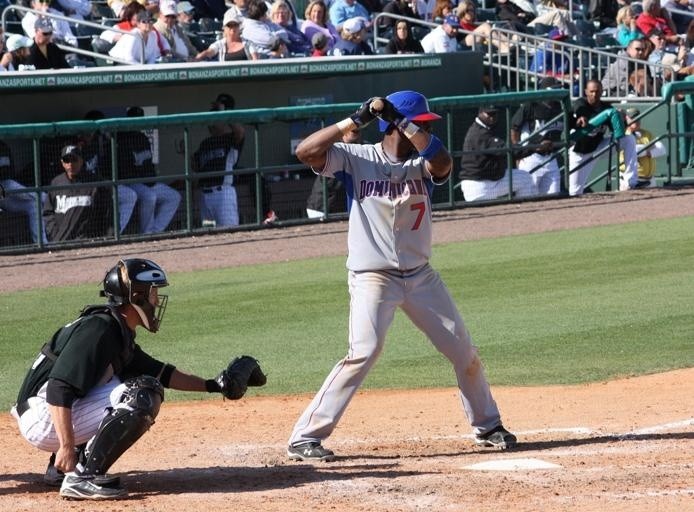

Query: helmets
<box><xmin>379</xmin><ymin>91</ymin><xmax>442</xmax><ymax>132</ymax></box>
<box><xmin>99</xmin><ymin>258</ymin><xmax>169</xmax><ymax>333</ymax></box>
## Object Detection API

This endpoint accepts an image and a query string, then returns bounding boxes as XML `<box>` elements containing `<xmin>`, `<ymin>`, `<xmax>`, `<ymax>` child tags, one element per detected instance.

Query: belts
<box><xmin>202</xmin><ymin>186</ymin><xmax>221</xmax><ymax>193</ymax></box>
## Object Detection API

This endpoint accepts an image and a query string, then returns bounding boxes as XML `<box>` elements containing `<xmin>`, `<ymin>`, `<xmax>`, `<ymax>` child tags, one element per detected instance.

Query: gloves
<box><xmin>351</xmin><ymin>97</ymin><xmax>409</xmax><ymax>131</ymax></box>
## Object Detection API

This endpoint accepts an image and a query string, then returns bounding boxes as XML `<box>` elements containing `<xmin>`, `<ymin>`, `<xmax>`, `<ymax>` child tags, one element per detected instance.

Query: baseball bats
<box><xmin>374</xmin><ymin>99</ymin><xmax>384</xmax><ymax>111</ymax></box>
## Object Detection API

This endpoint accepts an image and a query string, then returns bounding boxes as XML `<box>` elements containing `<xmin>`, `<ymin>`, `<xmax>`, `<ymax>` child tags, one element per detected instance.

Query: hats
<box><xmin>539</xmin><ymin>78</ymin><xmax>562</xmax><ymax>88</ymax></box>
<box><xmin>223</xmin><ymin>8</ymin><xmax>240</xmax><ymax>25</ymax></box>
<box><xmin>61</xmin><ymin>145</ymin><xmax>81</xmax><ymax>157</ymax></box>
<box><xmin>136</xmin><ymin>0</ymin><xmax>195</xmax><ymax>21</ymax></box>
<box><xmin>212</xmin><ymin>94</ymin><xmax>234</xmax><ymax>109</ymax></box>
<box><xmin>626</xmin><ymin>108</ymin><xmax>639</xmax><ymax>118</ymax></box>
<box><xmin>35</xmin><ymin>18</ymin><xmax>54</xmax><ymax>33</ymax></box>
<box><xmin>444</xmin><ymin>15</ymin><xmax>461</xmax><ymax>27</ymax></box>
<box><xmin>343</xmin><ymin>18</ymin><xmax>364</xmax><ymax>33</ymax></box>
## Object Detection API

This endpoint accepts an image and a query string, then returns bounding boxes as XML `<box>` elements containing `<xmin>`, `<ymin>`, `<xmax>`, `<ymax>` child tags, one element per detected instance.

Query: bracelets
<box><xmin>416</xmin><ymin>131</ymin><xmax>441</xmax><ymax>162</ymax></box>
<box><xmin>159</xmin><ymin>361</ymin><xmax>176</xmax><ymax>389</ymax></box>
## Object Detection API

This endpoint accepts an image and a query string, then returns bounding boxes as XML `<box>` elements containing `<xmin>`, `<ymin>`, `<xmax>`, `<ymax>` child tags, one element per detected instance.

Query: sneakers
<box><xmin>475</xmin><ymin>426</ymin><xmax>517</xmax><ymax>449</ymax></box>
<box><xmin>44</xmin><ymin>464</ymin><xmax>129</xmax><ymax>500</ymax></box>
<box><xmin>287</xmin><ymin>442</ymin><xmax>334</xmax><ymax>462</ymax></box>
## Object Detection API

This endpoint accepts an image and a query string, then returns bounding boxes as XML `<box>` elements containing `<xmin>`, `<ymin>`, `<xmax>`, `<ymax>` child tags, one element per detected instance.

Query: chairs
<box><xmin>68</xmin><ymin>7</ymin><xmax>225</xmax><ymax>68</ymax></box>
<box><xmin>455</xmin><ymin>10</ymin><xmax>617</xmax><ymax>80</ymax></box>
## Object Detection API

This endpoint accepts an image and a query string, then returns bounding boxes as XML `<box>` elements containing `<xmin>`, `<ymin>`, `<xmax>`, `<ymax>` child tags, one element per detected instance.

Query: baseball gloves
<box><xmin>216</xmin><ymin>355</ymin><xmax>266</xmax><ymax>400</ymax></box>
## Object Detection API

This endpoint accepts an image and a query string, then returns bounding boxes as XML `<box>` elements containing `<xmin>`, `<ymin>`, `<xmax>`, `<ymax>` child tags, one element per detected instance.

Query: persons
<box><xmin>306</xmin><ymin>127</ymin><xmax>370</xmax><ymax>218</ymax></box>
<box><xmin>0</xmin><ymin>0</ymin><xmax>694</xmax><ymax>98</ymax></box>
<box><xmin>286</xmin><ymin>89</ymin><xmax>517</xmax><ymax>464</ymax></box>
<box><xmin>0</xmin><ymin>93</ymin><xmax>246</xmax><ymax>246</ymax></box>
<box><xmin>10</xmin><ymin>255</ymin><xmax>268</xmax><ymax>499</ymax></box>
<box><xmin>459</xmin><ymin>81</ymin><xmax>666</xmax><ymax>201</ymax></box>
